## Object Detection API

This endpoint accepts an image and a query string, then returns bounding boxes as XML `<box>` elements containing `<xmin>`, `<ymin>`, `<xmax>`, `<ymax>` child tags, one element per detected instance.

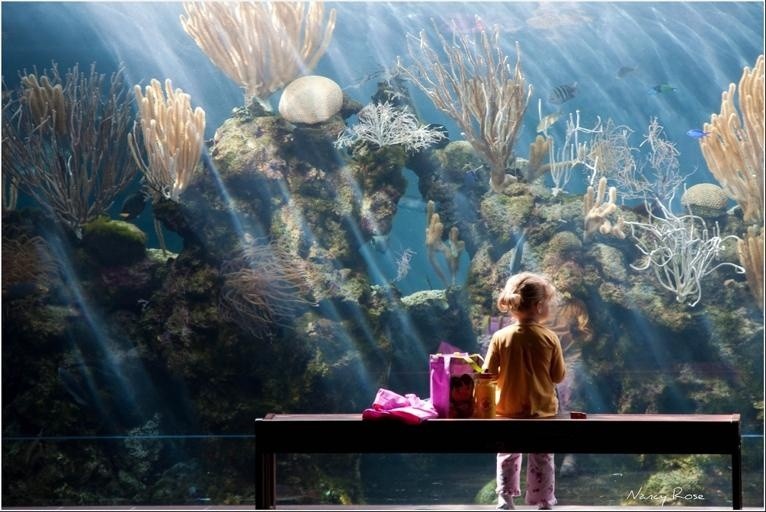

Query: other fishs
<box><xmin>654</xmin><ymin>82</ymin><xmax>677</xmax><ymax>93</ymax></box>
<box><xmin>686</xmin><ymin>128</ymin><xmax>710</xmax><ymax>140</ymax></box>
<box><xmin>616</xmin><ymin>65</ymin><xmax>640</xmax><ymax>78</ymax></box>
<box><xmin>549</xmin><ymin>81</ymin><xmax>581</xmax><ymax>105</ymax></box>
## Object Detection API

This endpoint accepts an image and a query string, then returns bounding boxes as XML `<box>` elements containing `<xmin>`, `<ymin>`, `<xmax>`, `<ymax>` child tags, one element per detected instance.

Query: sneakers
<box><xmin>538</xmin><ymin>500</ymin><xmax>554</xmax><ymax>510</ymax></box>
<box><xmin>496</xmin><ymin>495</ymin><xmax>515</xmax><ymax>509</ymax></box>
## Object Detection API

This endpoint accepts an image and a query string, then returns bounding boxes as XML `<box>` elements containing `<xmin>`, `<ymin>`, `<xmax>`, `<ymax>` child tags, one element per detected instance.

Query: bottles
<box><xmin>475</xmin><ymin>371</ymin><xmax>496</xmax><ymax>418</ymax></box>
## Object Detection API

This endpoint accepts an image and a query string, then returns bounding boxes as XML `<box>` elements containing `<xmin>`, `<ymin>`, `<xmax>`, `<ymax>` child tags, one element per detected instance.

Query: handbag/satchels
<box><xmin>429</xmin><ymin>351</ymin><xmax>484</xmax><ymax>418</ymax></box>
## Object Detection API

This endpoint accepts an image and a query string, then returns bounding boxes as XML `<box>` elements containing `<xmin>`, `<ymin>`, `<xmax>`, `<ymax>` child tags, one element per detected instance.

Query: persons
<box><xmin>478</xmin><ymin>271</ymin><xmax>566</xmax><ymax>509</ymax></box>
<box><xmin>448</xmin><ymin>373</ymin><xmax>474</xmax><ymax>419</ymax></box>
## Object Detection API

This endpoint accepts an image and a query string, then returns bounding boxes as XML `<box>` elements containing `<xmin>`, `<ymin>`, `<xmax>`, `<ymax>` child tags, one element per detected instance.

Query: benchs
<box><xmin>253</xmin><ymin>412</ymin><xmax>744</xmax><ymax>510</ymax></box>
